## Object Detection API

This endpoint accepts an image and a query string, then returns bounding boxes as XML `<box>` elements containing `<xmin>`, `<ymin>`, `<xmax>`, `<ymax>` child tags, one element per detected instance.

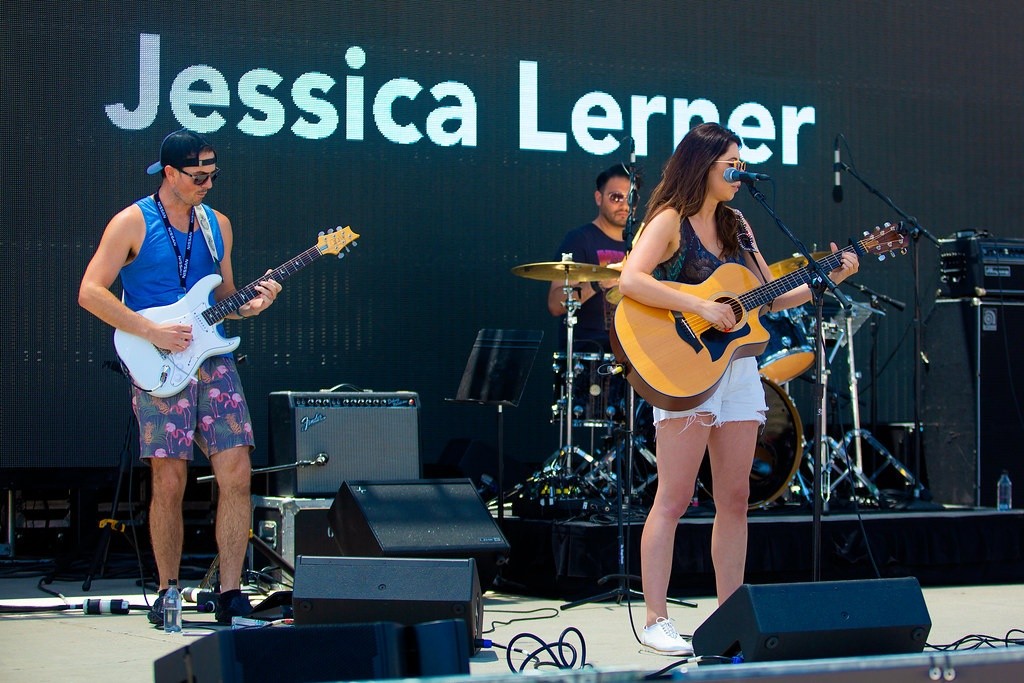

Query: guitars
<box><xmin>113</xmin><ymin>225</ymin><xmax>361</xmax><ymax>398</ymax></box>
<box><xmin>608</xmin><ymin>221</ymin><xmax>913</xmax><ymax>412</ymax></box>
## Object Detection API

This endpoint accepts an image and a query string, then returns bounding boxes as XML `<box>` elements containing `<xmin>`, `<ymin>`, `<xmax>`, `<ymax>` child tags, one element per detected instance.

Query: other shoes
<box><xmin>148</xmin><ymin>592</ymin><xmax>182</xmax><ymax>625</ymax></box>
<box><xmin>216</xmin><ymin>592</ymin><xmax>254</xmax><ymax>625</ymax></box>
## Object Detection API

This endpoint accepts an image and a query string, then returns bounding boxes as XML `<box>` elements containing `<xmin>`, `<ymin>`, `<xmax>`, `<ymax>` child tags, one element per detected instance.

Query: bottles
<box><xmin>997</xmin><ymin>470</ymin><xmax>1013</xmax><ymax>511</ymax></box>
<box><xmin>163</xmin><ymin>578</ymin><xmax>183</xmax><ymax>632</ymax></box>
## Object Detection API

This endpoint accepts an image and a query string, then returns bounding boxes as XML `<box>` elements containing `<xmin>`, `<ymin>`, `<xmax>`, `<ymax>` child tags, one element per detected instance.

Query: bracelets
<box><xmin>235</xmin><ymin>309</ymin><xmax>249</xmax><ymax>321</ymax></box>
<box><xmin>590</xmin><ymin>282</ymin><xmax>605</xmax><ymax>294</ymax></box>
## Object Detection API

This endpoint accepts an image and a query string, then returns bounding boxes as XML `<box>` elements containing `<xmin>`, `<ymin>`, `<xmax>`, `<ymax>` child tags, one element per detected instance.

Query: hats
<box><xmin>146</xmin><ymin>128</ymin><xmax>218</xmax><ymax>176</ymax></box>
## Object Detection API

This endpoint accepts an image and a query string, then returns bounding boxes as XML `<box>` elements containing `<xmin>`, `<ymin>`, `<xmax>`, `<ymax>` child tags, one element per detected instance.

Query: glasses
<box><xmin>173</xmin><ymin>166</ymin><xmax>221</xmax><ymax>185</ymax></box>
<box><xmin>608</xmin><ymin>192</ymin><xmax>640</xmax><ymax>204</ymax></box>
<box><xmin>713</xmin><ymin>160</ymin><xmax>746</xmax><ymax>173</ymax></box>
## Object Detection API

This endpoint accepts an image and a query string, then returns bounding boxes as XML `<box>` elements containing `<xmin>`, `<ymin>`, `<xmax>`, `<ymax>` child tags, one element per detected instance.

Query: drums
<box><xmin>627</xmin><ymin>373</ymin><xmax>804</xmax><ymax>510</ymax></box>
<box><xmin>754</xmin><ymin>305</ymin><xmax>816</xmax><ymax>386</ymax></box>
<box><xmin>548</xmin><ymin>351</ymin><xmax>633</xmax><ymax>429</ymax></box>
<box><xmin>800</xmin><ymin>314</ymin><xmax>826</xmax><ymax>342</ymax></box>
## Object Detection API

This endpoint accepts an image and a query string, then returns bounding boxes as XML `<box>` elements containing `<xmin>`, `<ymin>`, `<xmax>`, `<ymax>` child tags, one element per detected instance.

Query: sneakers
<box><xmin>638</xmin><ymin>617</ymin><xmax>694</xmax><ymax>657</ymax></box>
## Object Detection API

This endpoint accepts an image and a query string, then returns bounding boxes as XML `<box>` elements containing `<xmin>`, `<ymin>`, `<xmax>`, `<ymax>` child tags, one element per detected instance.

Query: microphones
<box><xmin>833</xmin><ymin>135</ymin><xmax>844</xmax><ymax>204</ymax></box>
<box><xmin>626</xmin><ymin>140</ymin><xmax>638</xmax><ymax>206</ymax></box>
<box><xmin>722</xmin><ymin>167</ymin><xmax>772</xmax><ymax>181</ymax></box>
<box><xmin>315</xmin><ymin>452</ymin><xmax>329</xmax><ymax>465</ymax></box>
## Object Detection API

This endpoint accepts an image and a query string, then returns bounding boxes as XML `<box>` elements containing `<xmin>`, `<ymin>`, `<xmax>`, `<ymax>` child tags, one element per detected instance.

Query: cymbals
<box><xmin>605</xmin><ymin>285</ymin><xmax>623</xmax><ymax>305</ymax></box>
<box><xmin>768</xmin><ymin>251</ymin><xmax>832</xmax><ymax>282</ymax></box>
<box><xmin>509</xmin><ymin>261</ymin><xmax>622</xmax><ymax>282</ymax></box>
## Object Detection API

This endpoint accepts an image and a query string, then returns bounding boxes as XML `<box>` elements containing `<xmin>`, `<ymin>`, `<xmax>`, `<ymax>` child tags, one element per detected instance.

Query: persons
<box><xmin>77</xmin><ymin>130</ymin><xmax>282</xmax><ymax>626</ymax></box>
<box><xmin>619</xmin><ymin>122</ymin><xmax>860</xmax><ymax>657</ymax></box>
<box><xmin>548</xmin><ymin>165</ymin><xmax>655</xmax><ymax>479</ymax></box>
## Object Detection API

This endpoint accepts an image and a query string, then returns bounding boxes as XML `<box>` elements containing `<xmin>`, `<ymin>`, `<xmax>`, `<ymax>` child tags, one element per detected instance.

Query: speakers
<box><xmin>266</xmin><ymin>390</ymin><xmax>421</xmax><ymax>500</ymax></box>
<box><xmin>291</xmin><ymin>554</ymin><xmax>485</xmax><ymax>656</ymax></box>
<box><xmin>150</xmin><ymin>619</ymin><xmax>469</xmax><ymax>683</ymax></box>
<box><xmin>923</xmin><ymin>299</ymin><xmax>1024</xmax><ymax>510</ymax></box>
<box><xmin>691</xmin><ymin>577</ymin><xmax>932</xmax><ymax>666</ymax></box>
<box><xmin>326</xmin><ymin>477</ymin><xmax>512</xmax><ymax>597</ymax></box>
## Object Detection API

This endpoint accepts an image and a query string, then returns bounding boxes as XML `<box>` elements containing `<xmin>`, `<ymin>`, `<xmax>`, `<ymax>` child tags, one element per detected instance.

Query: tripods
<box><xmin>558</xmin><ymin>427</ymin><xmax>700</xmax><ymax>609</ymax></box>
<box><xmin>748</xmin><ymin>186</ymin><xmax>932</xmax><ymax>512</ymax></box>
<box><xmin>481</xmin><ymin>269</ymin><xmax>658</xmax><ymax>514</ymax></box>
<box><xmin>195</xmin><ymin>518</ymin><xmax>295</xmax><ymax>595</ymax></box>
